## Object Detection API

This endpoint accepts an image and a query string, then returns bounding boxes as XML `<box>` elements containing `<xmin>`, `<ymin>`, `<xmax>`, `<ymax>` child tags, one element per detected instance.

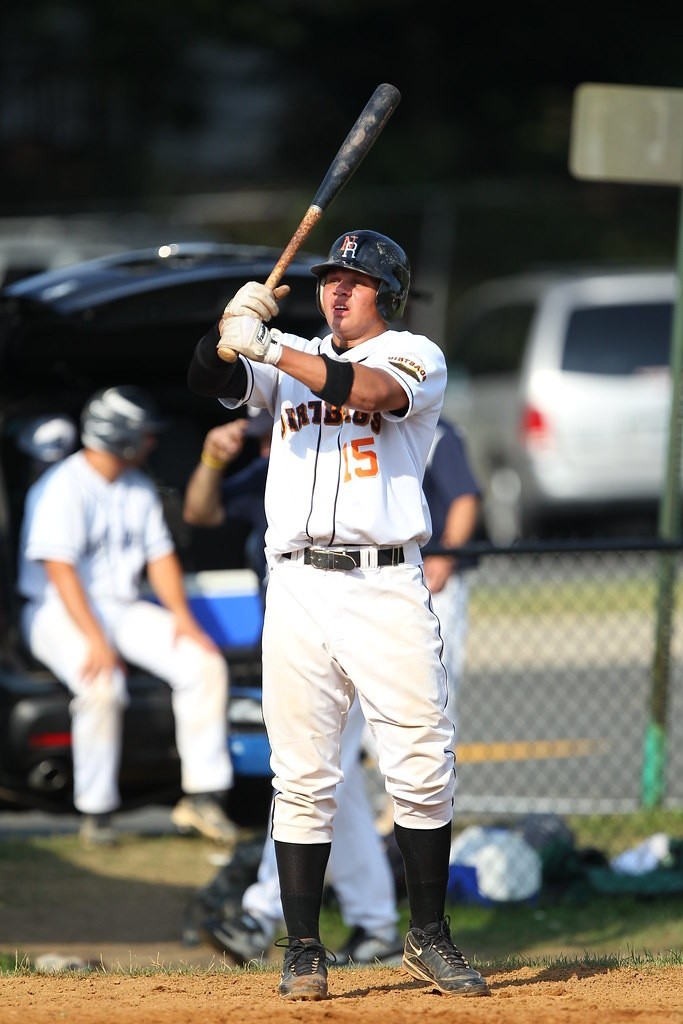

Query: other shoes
<box><xmin>79</xmin><ymin>816</ymin><xmax>115</xmax><ymax>853</ymax></box>
<box><xmin>210</xmin><ymin>911</ymin><xmax>270</xmax><ymax>969</ymax></box>
<box><xmin>171</xmin><ymin>794</ymin><xmax>256</xmax><ymax>845</ymax></box>
<box><xmin>329</xmin><ymin>925</ymin><xmax>404</xmax><ymax>963</ymax></box>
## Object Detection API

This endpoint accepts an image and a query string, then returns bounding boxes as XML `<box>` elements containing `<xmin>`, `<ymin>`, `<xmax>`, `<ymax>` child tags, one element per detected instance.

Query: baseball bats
<box><xmin>217</xmin><ymin>82</ymin><xmax>403</xmax><ymax>363</ymax></box>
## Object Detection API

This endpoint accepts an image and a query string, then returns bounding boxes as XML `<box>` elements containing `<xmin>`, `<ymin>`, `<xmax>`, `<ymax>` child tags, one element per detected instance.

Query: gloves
<box><xmin>222</xmin><ymin>280</ymin><xmax>289</xmax><ymax>323</ymax></box>
<box><xmin>217</xmin><ymin>315</ymin><xmax>283</xmax><ymax>365</ymax></box>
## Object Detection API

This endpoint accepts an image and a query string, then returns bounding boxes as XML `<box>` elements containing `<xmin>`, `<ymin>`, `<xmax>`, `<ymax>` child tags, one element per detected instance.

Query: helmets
<box><xmin>311</xmin><ymin>230</ymin><xmax>409</xmax><ymax>323</ymax></box>
<box><xmin>80</xmin><ymin>386</ymin><xmax>170</xmax><ymax>462</ymax></box>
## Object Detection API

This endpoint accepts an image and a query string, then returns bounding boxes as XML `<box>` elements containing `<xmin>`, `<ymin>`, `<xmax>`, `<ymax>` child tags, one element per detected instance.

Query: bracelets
<box><xmin>201</xmin><ymin>449</ymin><xmax>227</xmax><ymax>470</ymax></box>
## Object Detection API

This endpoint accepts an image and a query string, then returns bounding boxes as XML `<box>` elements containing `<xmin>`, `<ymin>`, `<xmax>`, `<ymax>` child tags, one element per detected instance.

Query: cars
<box><xmin>0</xmin><ymin>242</ymin><xmax>329</xmax><ymax>588</ymax></box>
<box><xmin>440</xmin><ymin>273</ymin><xmax>677</xmax><ymax>552</ymax></box>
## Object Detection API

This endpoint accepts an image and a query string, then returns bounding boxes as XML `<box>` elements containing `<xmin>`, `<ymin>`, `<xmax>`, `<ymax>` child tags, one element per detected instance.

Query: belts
<box><xmin>282</xmin><ymin>546</ymin><xmax>404</xmax><ymax>570</ymax></box>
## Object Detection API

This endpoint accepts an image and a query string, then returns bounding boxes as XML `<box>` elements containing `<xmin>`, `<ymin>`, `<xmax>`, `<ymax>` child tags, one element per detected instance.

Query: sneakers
<box><xmin>274</xmin><ymin>933</ymin><xmax>336</xmax><ymax>1002</ymax></box>
<box><xmin>401</xmin><ymin>911</ymin><xmax>491</xmax><ymax>998</ymax></box>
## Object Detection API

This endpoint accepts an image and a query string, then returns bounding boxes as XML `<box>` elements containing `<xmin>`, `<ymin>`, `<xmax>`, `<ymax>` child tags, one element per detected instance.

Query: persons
<box><xmin>422</xmin><ymin>419</ymin><xmax>485</xmax><ymax>681</ymax></box>
<box><xmin>185</xmin><ymin>419</ymin><xmax>403</xmax><ymax>966</ymax></box>
<box><xmin>17</xmin><ymin>387</ymin><xmax>237</xmax><ymax>844</ymax></box>
<box><xmin>192</xmin><ymin>229</ymin><xmax>492</xmax><ymax>1000</ymax></box>
